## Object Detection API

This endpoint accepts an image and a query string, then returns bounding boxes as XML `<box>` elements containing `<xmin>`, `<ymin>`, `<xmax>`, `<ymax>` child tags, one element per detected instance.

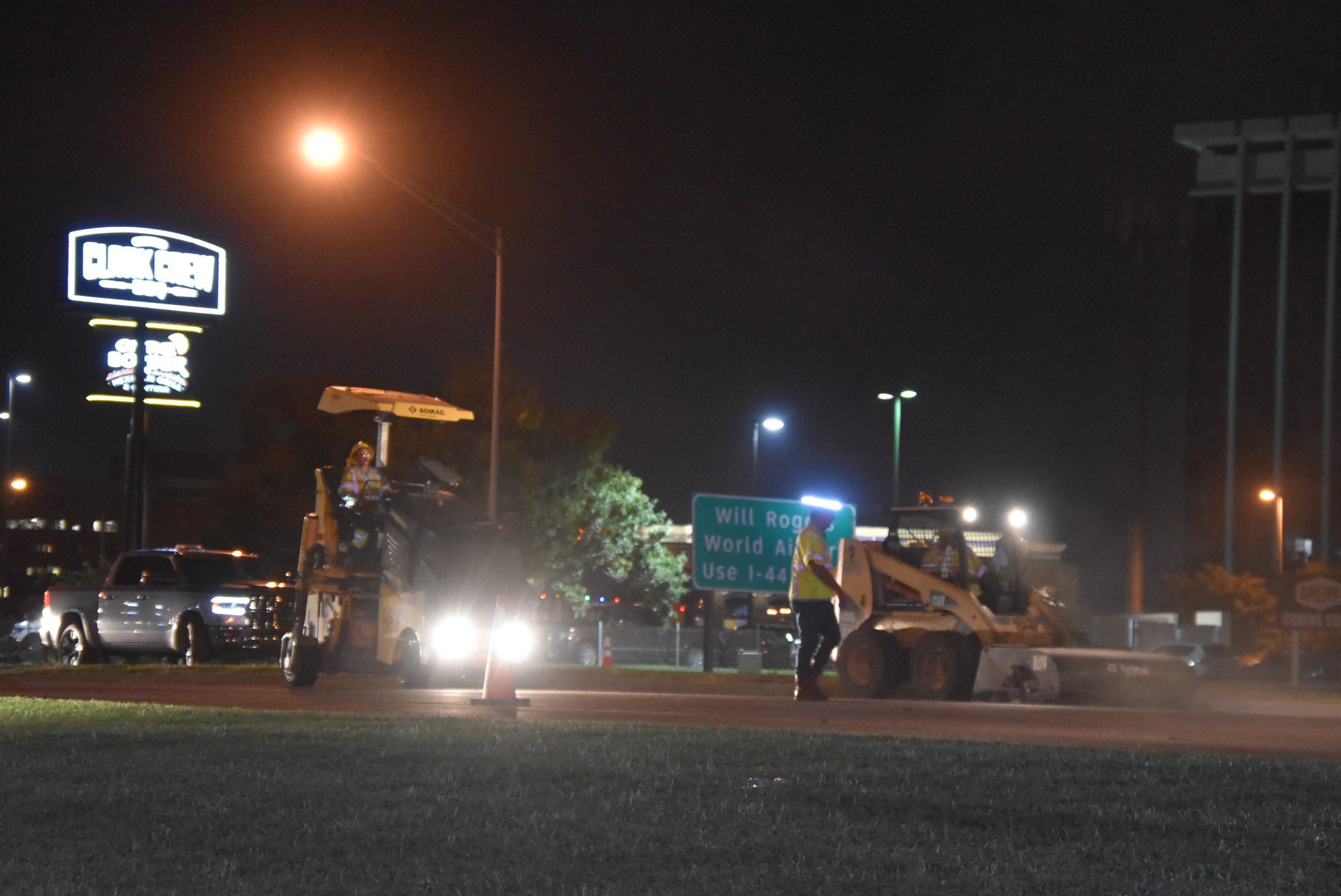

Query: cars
<box><xmin>2</xmin><ymin>605</ymin><xmax>40</xmax><ymax>662</ymax></box>
<box><xmin>1152</xmin><ymin>638</ymin><xmax>1236</xmax><ymax>680</ymax></box>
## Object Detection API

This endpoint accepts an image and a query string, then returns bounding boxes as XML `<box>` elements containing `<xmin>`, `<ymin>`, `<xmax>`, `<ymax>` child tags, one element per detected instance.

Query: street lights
<box><xmin>1256</xmin><ymin>489</ymin><xmax>1284</xmax><ymax>571</ymax></box>
<box><xmin>300</xmin><ymin>128</ymin><xmax>503</xmax><ymax>518</ymax></box>
<box><xmin>3</xmin><ymin>372</ymin><xmax>31</xmax><ymax>521</ymax></box>
<box><xmin>751</xmin><ymin>416</ymin><xmax>785</xmax><ymax>494</ymax></box>
<box><xmin>877</xmin><ymin>387</ymin><xmax>918</xmax><ymax>506</ymax></box>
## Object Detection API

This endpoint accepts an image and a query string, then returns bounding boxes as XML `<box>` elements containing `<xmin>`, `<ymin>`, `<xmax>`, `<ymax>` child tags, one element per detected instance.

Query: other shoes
<box><xmin>794</xmin><ymin>683</ymin><xmax>829</xmax><ymax>701</ymax></box>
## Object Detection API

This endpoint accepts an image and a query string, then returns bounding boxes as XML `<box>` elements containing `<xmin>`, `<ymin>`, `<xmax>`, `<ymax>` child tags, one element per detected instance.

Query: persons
<box><xmin>920</xmin><ymin>522</ymin><xmax>1016</xmax><ymax>613</ymax></box>
<box><xmin>337</xmin><ymin>441</ymin><xmax>399</xmax><ymax>567</ymax></box>
<box><xmin>788</xmin><ymin>506</ymin><xmax>847</xmax><ymax>702</ymax></box>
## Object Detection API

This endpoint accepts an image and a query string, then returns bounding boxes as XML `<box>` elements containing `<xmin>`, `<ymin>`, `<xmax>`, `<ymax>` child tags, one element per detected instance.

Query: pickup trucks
<box><xmin>41</xmin><ymin>546</ymin><xmax>298</xmax><ymax>668</ymax></box>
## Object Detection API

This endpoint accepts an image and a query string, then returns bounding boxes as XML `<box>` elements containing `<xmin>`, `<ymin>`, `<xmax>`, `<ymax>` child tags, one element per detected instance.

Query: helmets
<box><xmin>349</xmin><ymin>441</ymin><xmax>374</xmax><ymax>464</ymax></box>
<box><xmin>934</xmin><ymin>522</ymin><xmax>964</xmax><ymax>538</ymax></box>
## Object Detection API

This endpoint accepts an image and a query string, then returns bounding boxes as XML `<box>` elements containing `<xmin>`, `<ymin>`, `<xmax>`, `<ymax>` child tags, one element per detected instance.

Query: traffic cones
<box><xmin>600</xmin><ymin>634</ymin><xmax>614</xmax><ymax>668</ymax></box>
<box><xmin>468</xmin><ymin>595</ymin><xmax>529</xmax><ymax>707</ymax></box>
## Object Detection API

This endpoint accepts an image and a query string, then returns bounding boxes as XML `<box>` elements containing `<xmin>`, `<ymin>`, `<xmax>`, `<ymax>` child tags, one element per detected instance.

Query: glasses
<box><xmin>360</xmin><ymin>453</ymin><xmax>371</xmax><ymax>458</ymax></box>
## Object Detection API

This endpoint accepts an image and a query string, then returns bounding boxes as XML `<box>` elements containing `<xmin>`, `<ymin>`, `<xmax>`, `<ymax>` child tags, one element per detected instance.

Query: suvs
<box><xmin>564</xmin><ymin>602</ymin><xmax>725</xmax><ymax>668</ymax></box>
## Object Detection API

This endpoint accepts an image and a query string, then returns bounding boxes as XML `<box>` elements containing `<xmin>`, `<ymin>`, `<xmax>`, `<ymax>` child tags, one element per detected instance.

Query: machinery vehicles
<box><xmin>280</xmin><ymin>381</ymin><xmax>543</xmax><ymax>692</ymax></box>
<box><xmin>835</xmin><ymin>489</ymin><xmax>1196</xmax><ymax>703</ymax></box>
